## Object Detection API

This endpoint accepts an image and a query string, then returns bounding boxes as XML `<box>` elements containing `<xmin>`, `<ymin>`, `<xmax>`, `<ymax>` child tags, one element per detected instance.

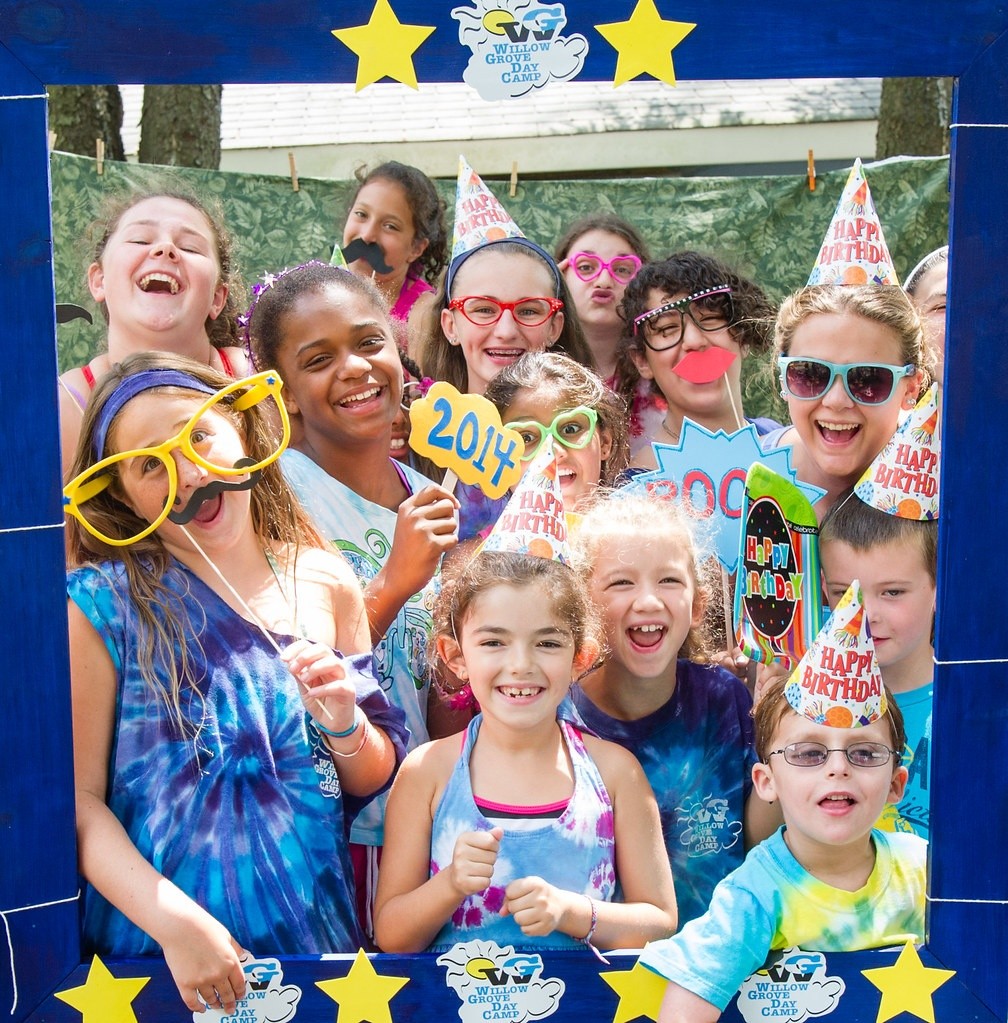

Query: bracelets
<box><xmin>314</xmin><ymin>714</ymin><xmax>360</xmax><ymax>737</ymax></box>
<box><xmin>321</xmin><ymin>708</ymin><xmax>369</xmax><ymax>757</ymax></box>
<box><xmin>430</xmin><ymin>667</ymin><xmax>473</xmax><ymax>710</ymax></box>
<box><xmin>571</xmin><ymin>894</ymin><xmax>610</xmax><ymax>964</ymax></box>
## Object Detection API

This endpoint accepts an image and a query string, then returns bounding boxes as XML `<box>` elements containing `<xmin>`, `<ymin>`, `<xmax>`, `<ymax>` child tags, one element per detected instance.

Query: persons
<box><xmin>58</xmin><ymin>193</ymin><xmax>256</xmax><ymax>568</ymax></box>
<box><xmin>422</xmin><ymin>237</ymin><xmax>597</xmax><ymax>545</ymax></box>
<box><xmin>759</xmin><ymin>284</ymin><xmax>924</xmax><ymax>527</ymax></box>
<box><xmin>67</xmin><ymin>351</ymin><xmax>411</xmax><ymax>1014</ymax></box>
<box><xmin>342</xmin><ymin>160</ymin><xmax>452</xmax><ymax>389</ymax></box>
<box><xmin>638</xmin><ymin>670</ymin><xmax>928</xmax><ymax>1023</ymax></box>
<box><xmin>904</xmin><ymin>245</ymin><xmax>948</xmax><ymax>389</ymax></box>
<box><xmin>373</xmin><ymin>551</ymin><xmax>678</xmax><ymax>951</ymax></box>
<box><xmin>442</xmin><ymin>353</ymin><xmax>630</xmax><ymax>588</ymax></box>
<box><xmin>554</xmin><ymin>214</ymin><xmax>667</xmax><ymax>456</ymax></box>
<box><xmin>817</xmin><ymin>484</ymin><xmax>938</xmax><ymax>845</ymax></box>
<box><xmin>390</xmin><ymin>355</ymin><xmax>447</xmax><ymax>486</ymax></box>
<box><xmin>562</xmin><ymin>494</ymin><xmax>785</xmax><ymax>933</ymax></box>
<box><xmin>615</xmin><ymin>252</ymin><xmax>786</xmax><ymax>487</ymax></box>
<box><xmin>241</xmin><ymin>261</ymin><xmax>472</xmax><ymax>950</ymax></box>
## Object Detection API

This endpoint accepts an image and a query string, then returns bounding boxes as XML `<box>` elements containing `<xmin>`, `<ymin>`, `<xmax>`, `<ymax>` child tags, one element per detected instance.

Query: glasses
<box><xmin>505</xmin><ymin>404</ymin><xmax>599</xmax><ymax>462</ymax></box>
<box><xmin>634</xmin><ymin>285</ymin><xmax>733</xmax><ymax>351</ymax></box>
<box><xmin>566</xmin><ymin>252</ymin><xmax>643</xmax><ymax>283</ymax></box>
<box><xmin>449</xmin><ymin>295</ymin><xmax>563</xmax><ymax>326</ymax></box>
<box><xmin>61</xmin><ymin>369</ymin><xmax>291</xmax><ymax>547</ymax></box>
<box><xmin>764</xmin><ymin>741</ymin><xmax>900</xmax><ymax>804</ymax></box>
<box><xmin>777</xmin><ymin>352</ymin><xmax>914</xmax><ymax>406</ymax></box>
